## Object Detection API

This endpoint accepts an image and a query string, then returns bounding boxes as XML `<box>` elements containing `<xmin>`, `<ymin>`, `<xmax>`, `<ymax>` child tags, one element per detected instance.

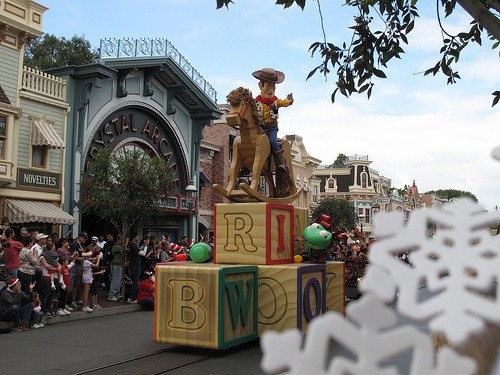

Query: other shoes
<box><xmin>116</xmin><ymin>295</ymin><xmax>122</xmax><ymax>298</ymax></box>
<box><xmin>108</xmin><ymin>296</ymin><xmax>118</xmax><ymax>301</ymax></box>
<box><xmin>13</xmin><ymin>325</ymin><xmax>28</xmax><ymax>332</ymax></box>
<box><xmin>31</xmin><ymin>324</ymin><xmax>40</xmax><ymax>328</ymax></box>
<box><xmin>128</xmin><ymin>298</ymin><xmax>137</xmax><ymax>303</ymax></box>
<box><xmin>91</xmin><ymin>304</ymin><xmax>101</xmax><ymax>310</ymax></box>
<box><xmin>51</xmin><ymin>285</ymin><xmax>56</xmax><ymax>290</ymax></box>
<box><xmin>60</xmin><ymin>281</ymin><xmax>66</xmax><ymax>288</ymax></box>
<box><xmin>38</xmin><ymin>323</ymin><xmax>44</xmax><ymax>327</ymax></box>
<box><xmin>72</xmin><ymin>302</ymin><xmax>77</xmax><ymax>307</ymax></box>
<box><xmin>47</xmin><ymin>312</ymin><xmax>53</xmax><ymax>317</ymax></box>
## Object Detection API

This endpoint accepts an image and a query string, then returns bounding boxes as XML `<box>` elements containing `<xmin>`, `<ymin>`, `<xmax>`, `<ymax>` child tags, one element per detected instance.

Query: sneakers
<box><xmin>65</xmin><ymin>305</ymin><xmax>73</xmax><ymax>310</ymax></box>
<box><xmin>63</xmin><ymin>308</ymin><xmax>71</xmax><ymax>314</ymax></box>
<box><xmin>83</xmin><ymin>306</ymin><xmax>93</xmax><ymax>312</ymax></box>
<box><xmin>56</xmin><ymin>309</ymin><xmax>65</xmax><ymax>315</ymax></box>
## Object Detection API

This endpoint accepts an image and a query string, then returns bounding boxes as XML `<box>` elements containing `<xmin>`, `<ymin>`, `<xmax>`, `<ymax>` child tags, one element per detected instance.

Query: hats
<box><xmin>8</xmin><ymin>278</ymin><xmax>18</xmax><ymax>287</ymax></box>
<box><xmin>36</xmin><ymin>233</ymin><xmax>48</xmax><ymax>240</ymax></box>
<box><xmin>92</xmin><ymin>236</ymin><xmax>98</xmax><ymax>241</ymax></box>
<box><xmin>78</xmin><ymin>232</ymin><xmax>89</xmax><ymax>238</ymax></box>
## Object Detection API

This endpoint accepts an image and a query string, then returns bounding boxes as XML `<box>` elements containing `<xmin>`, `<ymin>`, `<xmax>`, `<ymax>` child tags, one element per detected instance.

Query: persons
<box><xmin>239</xmin><ymin>68</ymin><xmax>293</xmax><ymax>173</ymax></box>
<box><xmin>0</xmin><ymin>217</ymin><xmax>195</xmax><ymax>331</ymax></box>
<box><xmin>328</xmin><ymin>244</ymin><xmax>346</xmax><ymax>261</ymax></box>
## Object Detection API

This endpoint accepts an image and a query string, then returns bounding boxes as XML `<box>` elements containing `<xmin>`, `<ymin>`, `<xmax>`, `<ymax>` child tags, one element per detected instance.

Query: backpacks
<box><xmin>105</xmin><ymin>244</ymin><xmax>119</xmax><ymax>261</ymax></box>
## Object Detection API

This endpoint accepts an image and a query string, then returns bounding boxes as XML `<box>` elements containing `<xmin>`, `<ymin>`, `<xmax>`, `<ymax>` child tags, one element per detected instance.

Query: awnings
<box><xmin>33</xmin><ymin>120</ymin><xmax>65</xmax><ymax>149</ymax></box>
<box><xmin>297</xmin><ymin>181</ymin><xmax>313</xmax><ymax>192</ymax></box>
<box><xmin>4</xmin><ymin>199</ymin><xmax>76</xmax><ymax>225</ymax></box>
